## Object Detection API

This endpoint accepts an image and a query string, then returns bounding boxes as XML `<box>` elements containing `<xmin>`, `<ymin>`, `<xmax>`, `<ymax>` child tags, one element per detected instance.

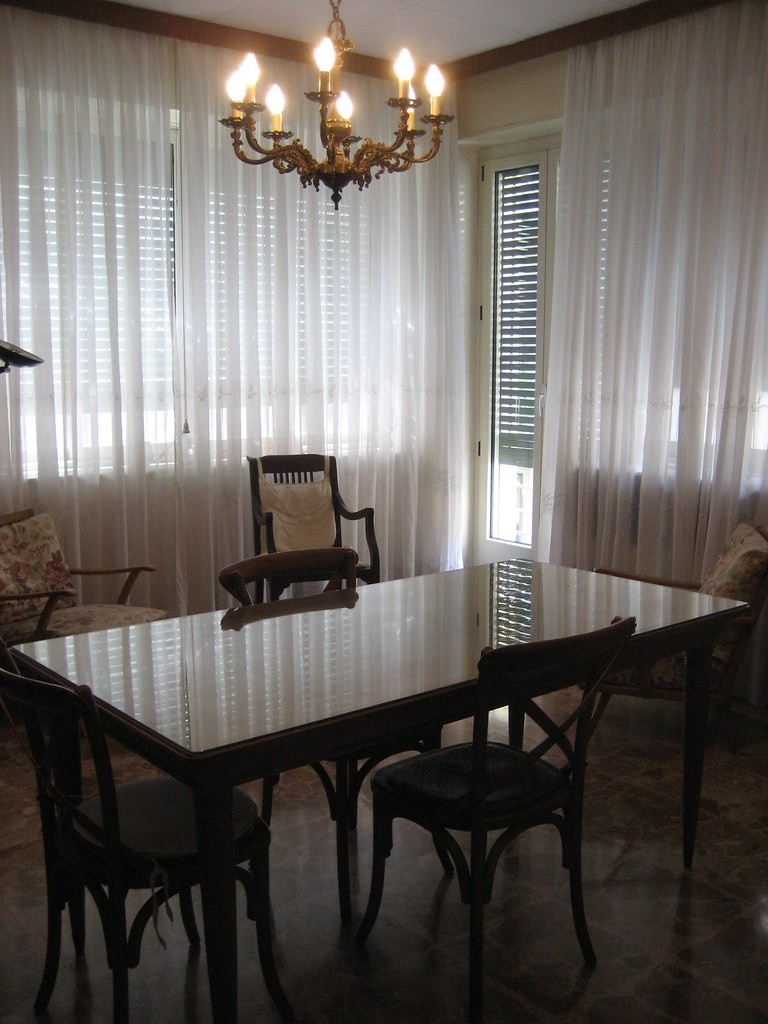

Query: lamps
<box><xmin>219</xmin><ymin>0</ymin><xmax>453</xmax><ymax>215</ymax></box>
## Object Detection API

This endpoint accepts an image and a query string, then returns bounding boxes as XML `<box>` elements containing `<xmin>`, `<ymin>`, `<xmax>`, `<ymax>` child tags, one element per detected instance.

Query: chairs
<box><xmin>568</xmin><ymin>522</ymin><xmax>768</xmax><ymax>766</ymax></box>
<box><xmin>356</xmin><ymin>617</ymin><xmax>642</xmax><ymax>1024</ymax></box>
<box><xmin>1</xmin><ymin>512</ymin><xmax>170</xmax><ymax>667</ymax></box>
<box><xmin>3</xmin><ymin>669</ymin><xmax>296</xmax><ymax>1024</ymax></box>
<box><xmin>245</xmin><ymin>454</ymin><xmax>382</xmax><ymax>607</ymax></box>
<box><xmin>220</xmin><ymin>546</ymin><xmax>444</xmax><ymax>833</ymax></box>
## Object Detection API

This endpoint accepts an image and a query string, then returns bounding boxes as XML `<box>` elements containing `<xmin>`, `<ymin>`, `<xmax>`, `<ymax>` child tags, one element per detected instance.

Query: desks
<box><xmin>7</xmin><ymin>558</ymin><xmax>751</xmax><ymax>1024</ymax></box>
